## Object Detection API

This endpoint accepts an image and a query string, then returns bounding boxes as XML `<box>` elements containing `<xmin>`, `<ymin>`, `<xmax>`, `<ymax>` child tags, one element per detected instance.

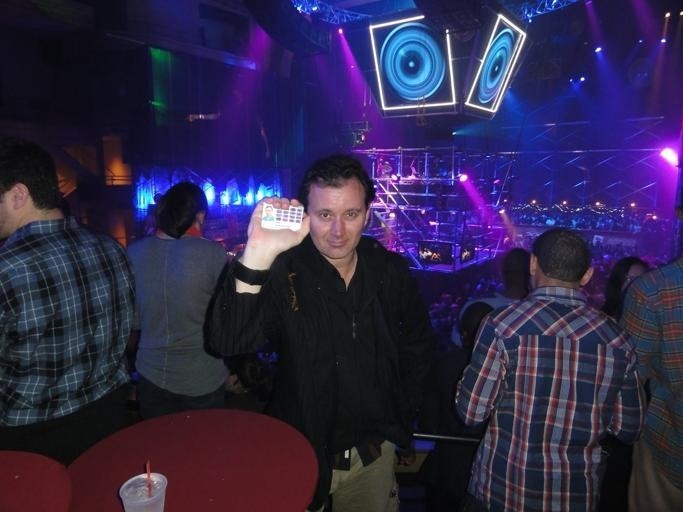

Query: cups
<box><xmin>118</xmin><ymin>473</ymin><xmax>167</xmax><ymax>511</ymax></box>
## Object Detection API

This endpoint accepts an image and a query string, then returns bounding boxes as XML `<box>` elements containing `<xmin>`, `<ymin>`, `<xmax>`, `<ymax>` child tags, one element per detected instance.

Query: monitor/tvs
<box><xmin>461</xmin><ymin>245</ymin><xmax>474</xmax><ymax>262</ymax></box>
<box><xmin>418</xmin><ymin>241</ymin><xmax>452</xmax><ymax>264</ymax></box>
